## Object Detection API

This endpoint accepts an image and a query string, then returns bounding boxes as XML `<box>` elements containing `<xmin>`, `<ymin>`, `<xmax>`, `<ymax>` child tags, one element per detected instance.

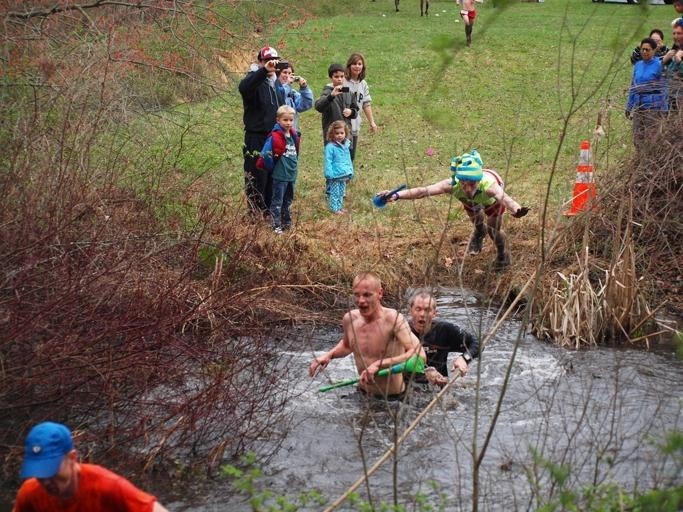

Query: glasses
<box><xmin>639</xmin><ymin>48</ymin><xmax>652</xmax><ymax>53</ymax></box>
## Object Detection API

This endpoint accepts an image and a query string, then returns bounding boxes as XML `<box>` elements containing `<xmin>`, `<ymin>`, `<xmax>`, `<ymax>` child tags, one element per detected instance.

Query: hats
<box><xmin>20</xmin><ymin>420</ymin><xmax>75</xmax><ymax>481</ymax></box>
<box><xmin>256</xmin><ymin>46</ymin><xmax>281</xmax><ymax>62</ymax></box>
<box><xmin>448</xmin><ymin>149</ymin><xmax>484</xmax><ymax>187</ymax></box>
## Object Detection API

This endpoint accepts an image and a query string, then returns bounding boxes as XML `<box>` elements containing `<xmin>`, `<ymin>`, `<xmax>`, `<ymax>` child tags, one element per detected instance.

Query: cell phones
<box><xmin>338</xmin><ymin>87</ymin><xmax>350</xmax><ymax>93</ymax></box>
<box><xmin>275</xmin><ymin>63</ymin><xmax>289</xmax><ymax>70</ymax></box>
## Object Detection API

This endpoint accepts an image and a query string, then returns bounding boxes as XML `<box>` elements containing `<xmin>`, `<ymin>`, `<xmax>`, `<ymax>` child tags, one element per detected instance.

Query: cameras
<box><xmin>293</xmin><ymin>75</ymin><xmax>300</xmax><ymax>84</ymax></box>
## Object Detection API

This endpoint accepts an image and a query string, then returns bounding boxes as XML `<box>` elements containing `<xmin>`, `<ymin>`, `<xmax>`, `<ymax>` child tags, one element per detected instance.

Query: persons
<box><xmin>341</xmin><ymin>53</ymin><xmax>378</xmax><ymax>165</ymax></box>
<box><xmin>315</xmin><ymin>63</ymin><xmax>359</xmax><ymax>198</ymax></box>
<box><xmin>630</xmin><ymin>29</ymin><xmax>669</xmax><ymax>66</ymax></box>
<box><xmin>275</xmin><ymin>60</ymin><xmax>314</xmax><ymax>131</ymax></box>
<box><xmin>375</xmin><ymin>150</ymin><xmax>531</xmax><ymax>271</ymax></box>
<box><xmin>238</xmin><ymin>46</ymin><xmax>286</xmax><ymax>218</ymax></box>
<box><xmin>662</xmin><ymin>18</ymin><xmax>683</xmax><ymax>114</ymax></box>
<box><xmin>321</xmin><ymin>120</ymin><xmax>353</xmax><ymax>214</ymax></box>
<box><xmin>8</xmin><ymin>421</ymin><xmax>167</xmax><ymax>512</ymax></box>
<box><xmin>455</xmin><ymin>0</ymin><xmax>483</xmax><ymax>46</ymax></box>
<box><xmin>308</xmin><ymin>271</ymin><xmax>427</xmax><ymax>401</ymax></box>
<box><xmin>624</xmin><ymin>38</ymin><xmax>667</xmax><ymax>148</ymax></box>
<box><xmin>254</xmin><ymin>105</ymin><xmax>301</xmax><ymax>234</ymax></box>
<box><xmin>420</xmin><ymin>0</ymin><xmax>429</xmax><ymax>17</ymax></box>
<box><xmin>407</xmin><ymin>292</ymin><xmax>484</xmax><ymax>387</ymax></box>
<box><xmin>672</xmin><ymin>0</ymin><xmax>683</xmax><ymax>17</ymax></box>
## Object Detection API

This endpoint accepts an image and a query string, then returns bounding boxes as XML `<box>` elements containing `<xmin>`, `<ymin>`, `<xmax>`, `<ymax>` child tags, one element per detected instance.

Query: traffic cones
<box><xmin>561</xmin><ymin>140</ymin><xmax>608</xmax><ymax>216</ymax></box>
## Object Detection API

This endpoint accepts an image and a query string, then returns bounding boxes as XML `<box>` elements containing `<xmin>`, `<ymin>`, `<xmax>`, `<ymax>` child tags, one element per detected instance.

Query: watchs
<box><xmin>458</xmin><ymin>352</ymin><xmax>473</xmax><ymax>363</ymax></box>
